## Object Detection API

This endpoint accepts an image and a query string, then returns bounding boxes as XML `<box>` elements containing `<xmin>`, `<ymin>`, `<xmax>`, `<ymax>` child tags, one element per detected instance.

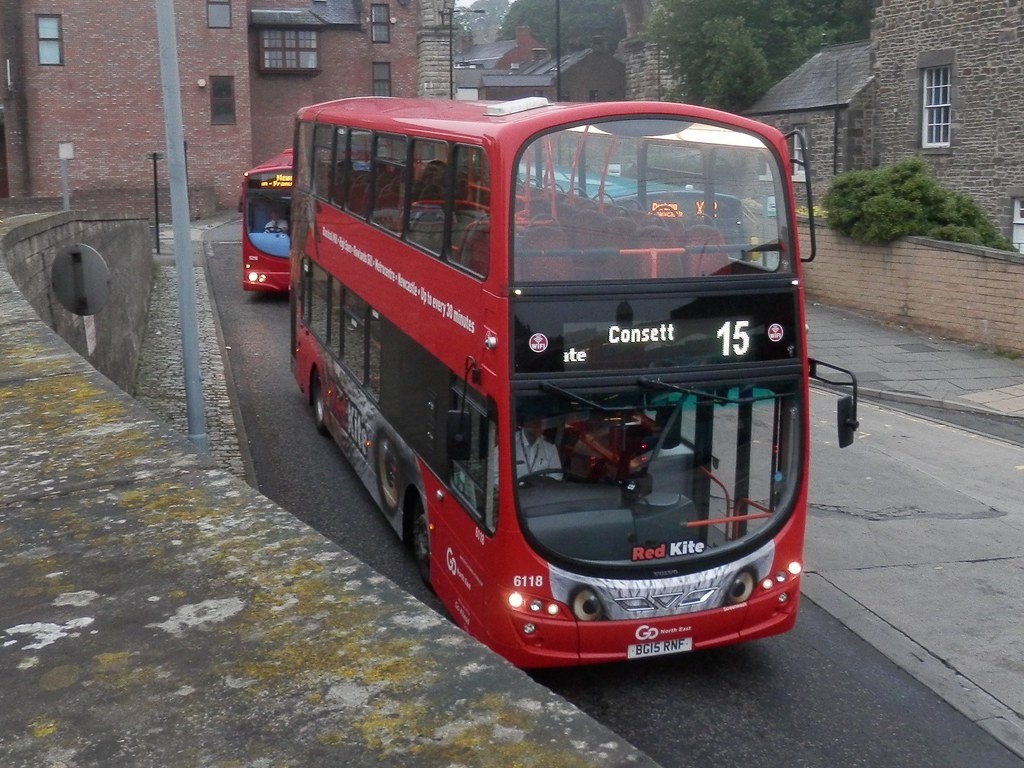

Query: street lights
<box><xmin>450</xmin><ymin>9</ymin><xmax>485</xmax><ymax>100</ymax></box>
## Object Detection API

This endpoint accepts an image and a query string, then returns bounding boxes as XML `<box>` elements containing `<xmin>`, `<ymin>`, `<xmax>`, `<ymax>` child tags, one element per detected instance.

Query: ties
<box><xmin>275</xmin><ymin>221</ymin><xmax>278</xmax><ymax>225</ymax></box>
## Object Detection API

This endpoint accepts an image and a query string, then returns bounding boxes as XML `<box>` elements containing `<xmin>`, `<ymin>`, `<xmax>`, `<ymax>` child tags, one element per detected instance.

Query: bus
<box><xmin>287</xmin><ymin>96</ymin><xmax>863</xmax><ymax>669</ymax></box>
<box><xmin>237</xmin><ymin>146</ymin><xmax>342</xmax><ymax>294</ymax></box>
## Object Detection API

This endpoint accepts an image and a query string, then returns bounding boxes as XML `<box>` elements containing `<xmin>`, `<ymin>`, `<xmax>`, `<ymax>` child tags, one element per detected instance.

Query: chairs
<box><xmin>319</xmin><ymin>156</ymin><xmax>734</xmax><ymax>282</ymax></box>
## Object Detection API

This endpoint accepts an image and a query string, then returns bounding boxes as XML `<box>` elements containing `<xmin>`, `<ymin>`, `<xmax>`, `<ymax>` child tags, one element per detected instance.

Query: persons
<box><xmin>489</xmin><ymin>412</ymin><xmax>563</xmax><ymax>488</ymax></box>
<box><xmin>263</xmin><ymin>210</ymin><xmax>288</xmax><ymax>233</ymax></box>
